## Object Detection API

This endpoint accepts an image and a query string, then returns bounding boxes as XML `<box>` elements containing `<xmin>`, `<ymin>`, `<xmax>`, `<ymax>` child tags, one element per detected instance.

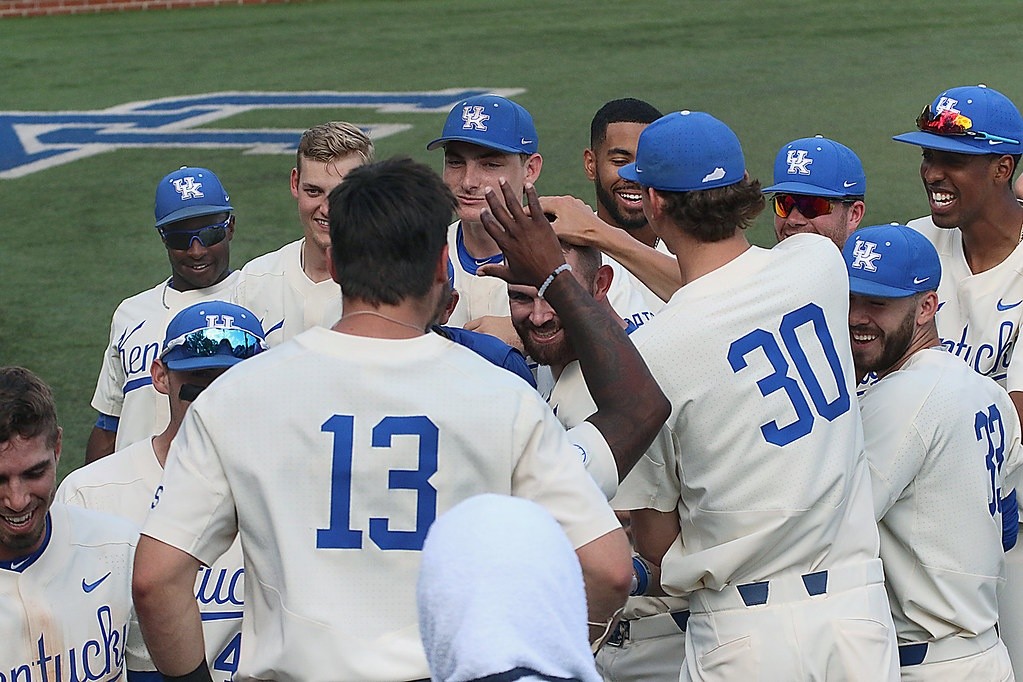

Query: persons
<box><xmin>0</xmin><ymin>84</ymin><xmax>1023</xmax><ymax>682</ymax></box>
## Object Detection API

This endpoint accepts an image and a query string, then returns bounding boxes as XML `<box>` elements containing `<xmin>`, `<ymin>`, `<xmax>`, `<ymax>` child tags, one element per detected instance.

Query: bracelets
<box><xmin>632</xmin><ymin>555</ymin><xmax>653</xmax><ymax>595</ymax></box>
<box><xmin>537</xmin><ymin>264</ymin><xmax>572</xmax><ymax>300</ymax></box>
<box><xmin>160</xmin><ymin>657</ymin><xmax>213</xmax><ymax>682</ymax></box>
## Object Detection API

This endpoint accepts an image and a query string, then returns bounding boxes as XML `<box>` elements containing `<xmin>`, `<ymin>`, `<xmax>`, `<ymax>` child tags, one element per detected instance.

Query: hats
<box><xmin>894</xmin><ymin>84</ymin><xmax>1023</xmax><ymax>154</ymax></box>
<box><xmin>162</xmin><ymin>301</ymin><xmax>265</xmax><ymax>371</ymax></box>
<box><xmin>761</xmin><ymin>135</ymin><xmax>867</xmax><ymax>200</ymax></box>
<box><xmin>842</xmin><ymin>223</ymin><xmax>942</xmax><ymax>297</ymax></box>
<box><xmin>155</xmin><ymin>167</ymin><xmax>234</xmax><ymax>229</ymax></box>
<box><xmin>427</xmin><ymin>96</ymin><xmax>539</xmax><ymax>155</ymax></box>
<box><xmin>618</xmin><ymin>111</ymin><xmax>746</xmax><ymax>193</ymax></box>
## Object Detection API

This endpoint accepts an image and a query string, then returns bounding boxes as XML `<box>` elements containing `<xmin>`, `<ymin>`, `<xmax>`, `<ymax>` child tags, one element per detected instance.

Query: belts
<box><xmin>898</xmin><ymin>622</ymin><xmax>1000</xmax><ymax>667</ymax></box>
<box><xmin>606</xmin><ymin>610</ymin><xmax>692</xmax><ymax>646</ymax></box>
<box><xmin>737</xmin><ymin>568</ymin><xmax>828</xmax><ymax>607</ymax></box>
<box><xmin>129</xmin><ymin>669</ymin><xmax>164</xmax><ymax>682</ymax></box>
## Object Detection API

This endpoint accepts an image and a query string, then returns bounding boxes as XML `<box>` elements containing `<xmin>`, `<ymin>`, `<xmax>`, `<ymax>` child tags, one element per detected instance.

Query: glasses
<box><xmin>160</xmin><ymin>215</ymin><xmax>231</xmax><ymax>251</ymax></box>
<box><xmin>770</xmin><ymin>193</ymin><xmax>856</xmax><ymax>220</ymax></box>
<box><xmin>914</xmin><ymin>105</ymin><xmax>1020</xmax><ymax>144</ymax></box>
<box><xmin>159</xmin><ymin>325</ymin><xmax>269</xmax><ymax>358</ymax></box>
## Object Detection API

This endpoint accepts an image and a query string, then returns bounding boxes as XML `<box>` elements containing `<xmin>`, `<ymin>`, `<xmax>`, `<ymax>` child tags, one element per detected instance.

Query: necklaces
<box><xmin>333</xmin><ymin>310</ymin><xmax>425</xmax><ymax>336</ymax></box>
